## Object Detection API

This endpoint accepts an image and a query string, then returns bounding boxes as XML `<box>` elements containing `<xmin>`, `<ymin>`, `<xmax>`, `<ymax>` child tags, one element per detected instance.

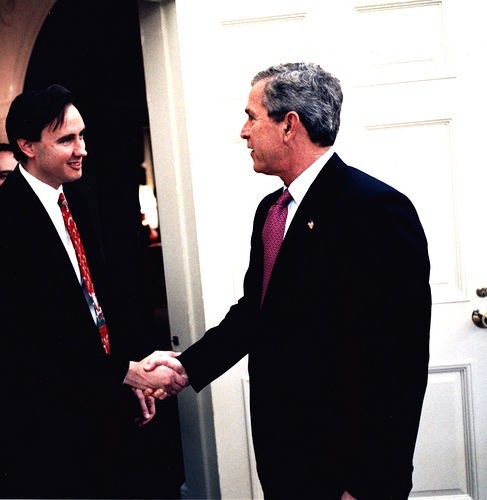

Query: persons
<box><xmin>143</xmin><ymin>61</ymin><xmax>432</xmax><ymax>500</ymax></box>
<box><xmin>0</xmin><ymin>84</ymin><xmax>188</xmax><ymax>500</ymax></box>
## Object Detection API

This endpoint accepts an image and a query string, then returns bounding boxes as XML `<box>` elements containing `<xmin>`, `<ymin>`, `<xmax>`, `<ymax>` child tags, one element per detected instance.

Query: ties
<box><xmin>261</xmin><ymin>189</ymin><xmax>293</xmax><ymax>307</ymax></box>
<box><xmin>58</xmin><ymin>193</ymin><xmax>109</xmax><ymax>355</ymax></box>
